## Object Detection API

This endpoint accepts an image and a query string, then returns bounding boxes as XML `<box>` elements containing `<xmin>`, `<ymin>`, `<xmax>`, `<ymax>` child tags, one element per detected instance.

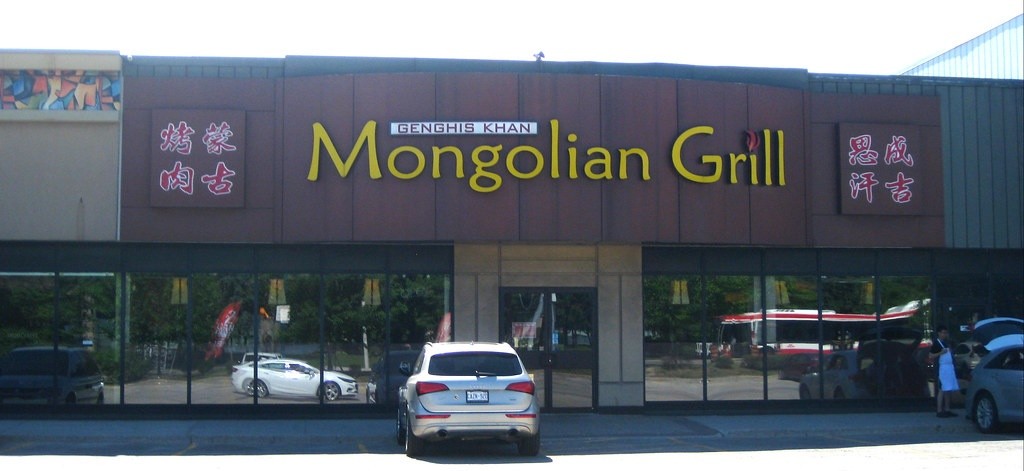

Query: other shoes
<box><xmin>937</xmin><ymin>412</ymin><xmax>949</xmax><ymax>418</ymax></box>
<box><xmin>949</xmin><ymin>412</ymin><xmax>957</xmax><ymax>416</ymax></box>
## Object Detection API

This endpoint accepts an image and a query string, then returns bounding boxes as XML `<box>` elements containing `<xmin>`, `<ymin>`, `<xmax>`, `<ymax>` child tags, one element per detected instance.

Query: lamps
<box><xmin>534</xmin><ymin>51</ymin><xmax>545</xmax><ymax>64</ymax></box>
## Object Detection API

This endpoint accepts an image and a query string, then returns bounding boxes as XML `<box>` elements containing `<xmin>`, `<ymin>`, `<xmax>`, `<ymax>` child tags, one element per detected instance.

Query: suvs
<box><xmin>365</xmin><ymin>342</ymin><xmax>421</xmax><ymax>411</ymax></box>
<box><xmin>395</xmin><ymin>340</ymin><xmax>542</xmax><ymax>457</ymax></box>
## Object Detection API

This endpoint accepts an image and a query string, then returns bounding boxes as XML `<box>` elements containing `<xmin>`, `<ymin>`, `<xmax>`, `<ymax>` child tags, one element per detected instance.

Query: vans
<box><xmin>0</xmin><ymin>345</ymin><xmax>106</xmax><ymax>409</ymax></box>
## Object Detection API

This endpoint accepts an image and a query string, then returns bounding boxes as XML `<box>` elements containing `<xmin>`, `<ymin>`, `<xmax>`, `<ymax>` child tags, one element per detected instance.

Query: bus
<box><xmin>714</xmin><ymin>295</ymin><xmax>932</xmax><ymax>368</ymax></box>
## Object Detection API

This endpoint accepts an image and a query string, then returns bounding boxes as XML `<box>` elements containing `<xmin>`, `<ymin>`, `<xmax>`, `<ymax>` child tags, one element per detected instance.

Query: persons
<box><xmin>929</xmin><ymin>327</ymin><xmax>959</xmax><ymax>416</ymax></box>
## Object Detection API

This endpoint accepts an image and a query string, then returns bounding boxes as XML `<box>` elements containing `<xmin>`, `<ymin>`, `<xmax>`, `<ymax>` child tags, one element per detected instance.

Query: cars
<box><xmin>798</xmin><ymin>324</ymin><xmax>933</xmax><ymax>414</ymax></box>
<box><xmin>230</xmin><ymin>351</ymin><xmax>359</xmax><ymax>401</ymax></box>
<box><xmin>964</xmin><ymin>316</ymin><xmax>1024</xmax><ymax>435</ymax></box>
<box><xmin>951</xmin><ymin>340</ymin><xmax>985</xmax><ymax>379</ymax></box>
